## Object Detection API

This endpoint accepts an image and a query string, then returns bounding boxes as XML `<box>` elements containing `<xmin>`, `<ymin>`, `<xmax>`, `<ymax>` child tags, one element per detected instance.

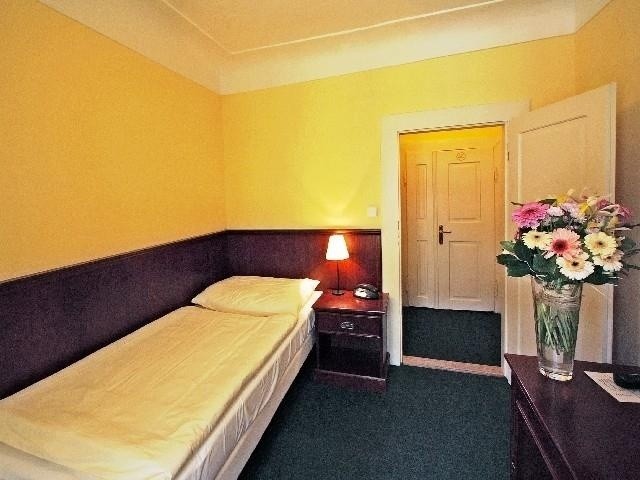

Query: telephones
<box><xmin>353</xmin><ymin>283</ymin><xmax>380</xmax><ymax>299</ymax></box>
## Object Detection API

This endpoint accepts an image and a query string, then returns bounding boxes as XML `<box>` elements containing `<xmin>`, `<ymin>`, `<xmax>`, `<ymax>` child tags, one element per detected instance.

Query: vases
<box><xmin>529</xmin><ymin>270</ymin><xmax>581</xmax><ymax>383</ymax></box>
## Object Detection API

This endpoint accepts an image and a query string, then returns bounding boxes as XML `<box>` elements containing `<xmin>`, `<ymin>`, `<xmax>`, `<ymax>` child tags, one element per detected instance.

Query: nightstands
<box><xmin>313</xmin><ymin>289</ymin><xmax>392</xmax><ymax>391</ymax></box>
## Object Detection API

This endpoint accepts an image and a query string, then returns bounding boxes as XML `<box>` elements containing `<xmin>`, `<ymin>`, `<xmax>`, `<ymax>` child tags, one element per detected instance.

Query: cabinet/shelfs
<box><xmin>504</xmin><ymin>355</ymin><xmax>640</xmax><ymax>478</ymax></box>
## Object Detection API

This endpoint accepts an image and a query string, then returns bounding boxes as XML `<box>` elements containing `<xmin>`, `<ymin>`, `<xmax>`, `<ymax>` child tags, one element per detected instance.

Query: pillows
<box><xmin>192</xmin><ymin>275</ymin><xmax>321</xmax><ymax>319</ymax></box>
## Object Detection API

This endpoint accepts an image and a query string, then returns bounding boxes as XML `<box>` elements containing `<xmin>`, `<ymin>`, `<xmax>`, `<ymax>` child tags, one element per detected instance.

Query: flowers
<box><xmin>493</xmin><ymin>189</ymin><xmax>640</xmax><ymax>352</ymax></box>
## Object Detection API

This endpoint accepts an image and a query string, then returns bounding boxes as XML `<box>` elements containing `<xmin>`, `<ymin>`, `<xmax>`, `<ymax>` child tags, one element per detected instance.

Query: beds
<box><xmin>0</xmin><ymin>275</ymin><xmax>319</xmax><ymax>479</ymax></box>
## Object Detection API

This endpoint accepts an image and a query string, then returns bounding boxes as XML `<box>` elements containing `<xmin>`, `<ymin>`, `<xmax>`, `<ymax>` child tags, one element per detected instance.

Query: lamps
<box><xmin>325</xmin><ymin>235</ymin><xmax>351</xmax><ymax>295</ymax></box>
